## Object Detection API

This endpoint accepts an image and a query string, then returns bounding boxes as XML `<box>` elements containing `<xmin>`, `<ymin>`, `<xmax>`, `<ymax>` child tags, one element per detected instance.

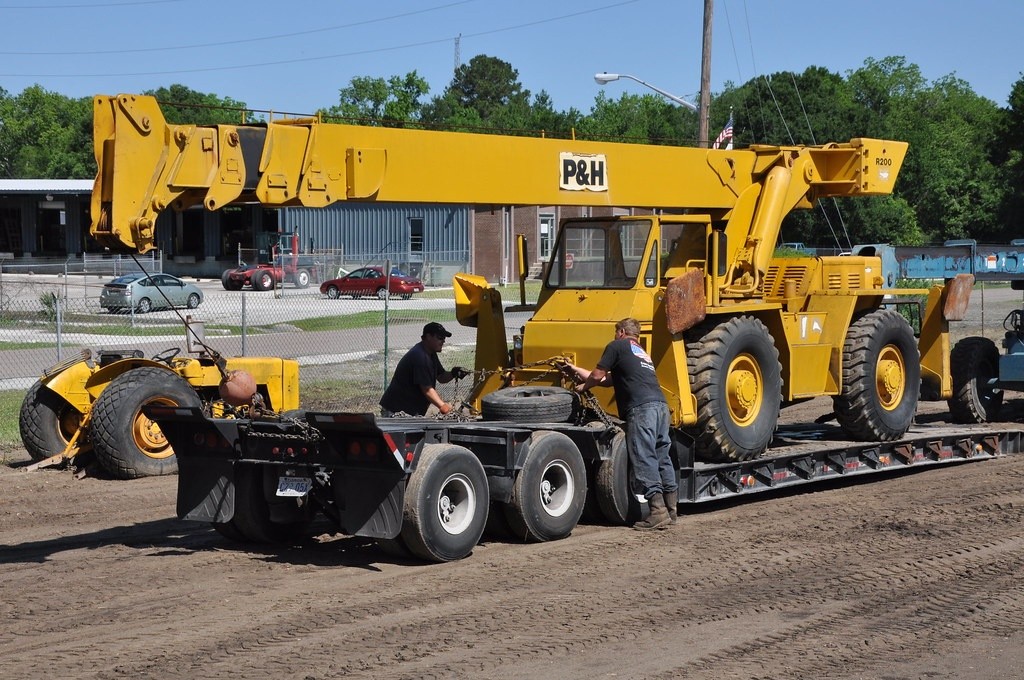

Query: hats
<box><xmin>421</xmin><ymin>322</ymin><xmax>452</xmax><ymax>339</ymax></box>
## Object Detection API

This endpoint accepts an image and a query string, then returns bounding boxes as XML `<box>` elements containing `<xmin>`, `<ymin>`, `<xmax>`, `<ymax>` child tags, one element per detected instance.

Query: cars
<box><xmin>100</xmin><ymin>271</ymin><xmax>204</xmax><ymax>314</ymax></box>
<box><xmin>320</xmin><ymin>266</ymin><xmax>425</xmax><ymax>300</ymax></box>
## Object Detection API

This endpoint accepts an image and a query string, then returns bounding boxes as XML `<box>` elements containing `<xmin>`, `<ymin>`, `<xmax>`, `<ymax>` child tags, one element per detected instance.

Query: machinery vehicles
<box><xmin>222</xmin><ymin>225</ymin><xmax>316</xmax><ymax>291</ymax></box>
<box><xmin>946</xmin><ymin>280</ymin><xmax>1023</xmax><ymax>423</ymax></box>
<box><xmin>19</xmin><ymin>314</ymin><xmax>299</xmax><ymax>480</ymax></box>
<box><xmin>88</xmin><ymin>94</ymin><xmax>976</xmax><ymax>464</ymax></box>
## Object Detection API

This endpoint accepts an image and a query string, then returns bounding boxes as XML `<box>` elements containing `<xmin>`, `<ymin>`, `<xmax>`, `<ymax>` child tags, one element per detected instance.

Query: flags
<box><xmin>713</xmin><ymin>120</ymin><xmax>733</xmax><ymax>149</ymax></box>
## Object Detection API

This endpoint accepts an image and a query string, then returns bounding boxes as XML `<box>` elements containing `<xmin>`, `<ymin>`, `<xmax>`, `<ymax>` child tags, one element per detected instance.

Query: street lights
<box><xmin>595</xmin><ymin>72</ymin><xmax>710</xmax><ymax>149</ymax></box>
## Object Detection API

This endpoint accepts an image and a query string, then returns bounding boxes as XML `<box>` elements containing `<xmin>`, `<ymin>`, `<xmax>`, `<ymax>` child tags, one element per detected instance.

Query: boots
<box><xmin>663</xmin><ymin>490</ymin><xmax>677</xmax><ymax>525</ymax></box>
<box><xmin>634</xmin><ymin>493</ymin><xmax>672</xmax><ymax>531</ymax></box>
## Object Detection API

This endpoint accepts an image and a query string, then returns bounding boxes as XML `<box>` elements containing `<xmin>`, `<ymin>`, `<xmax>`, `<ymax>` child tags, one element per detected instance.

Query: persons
<box><xmin>564</xmin><ymin>318</ymin><xmax>678</xmax><ymax>531</ymax></box>
<box><xmin>379</xmin><ymin>322</ymin><xmax>467</xmax><ymax>418</ymax></box>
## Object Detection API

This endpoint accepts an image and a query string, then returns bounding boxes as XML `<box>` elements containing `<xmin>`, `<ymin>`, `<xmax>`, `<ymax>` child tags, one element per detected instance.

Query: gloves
<box><xmin>451</xmin><ymin>367</ymin><xmax>469</xmax><ymax>382</ymax></box>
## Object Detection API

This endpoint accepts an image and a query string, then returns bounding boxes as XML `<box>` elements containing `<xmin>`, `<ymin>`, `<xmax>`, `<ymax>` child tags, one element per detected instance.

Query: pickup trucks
<box><xmin>777</xmin><ymin>242</ymin><xmax>817</xmax><ymax>258</ymax></box>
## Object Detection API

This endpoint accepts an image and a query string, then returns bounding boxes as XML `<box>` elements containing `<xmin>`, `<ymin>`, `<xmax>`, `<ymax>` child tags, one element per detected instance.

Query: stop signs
<box><xmin>566</xmin><ymin>253</ymin><xmax>574</xmax><ymax>270</ymax></box>
